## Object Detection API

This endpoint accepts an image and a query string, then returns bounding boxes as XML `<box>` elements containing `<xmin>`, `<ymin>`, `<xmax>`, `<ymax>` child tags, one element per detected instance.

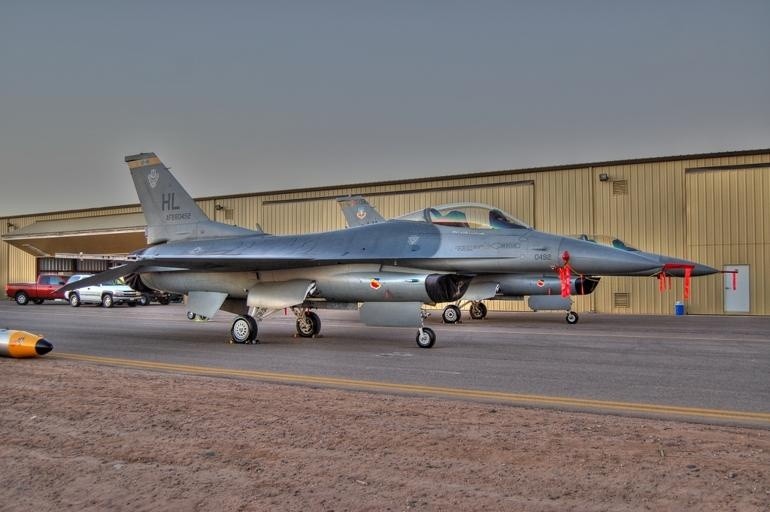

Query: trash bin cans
<box><xmin>674</xmin><ymin>300</ymin><xmax>685</xmax><ymax>315</ymax></box>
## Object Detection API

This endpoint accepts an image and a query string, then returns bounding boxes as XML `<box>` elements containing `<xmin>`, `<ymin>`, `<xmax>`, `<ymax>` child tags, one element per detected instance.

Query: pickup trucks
<box><xmin>6</xmin><ymin>273</ymin><xmax>70</xmax><ymax>305</ymax></box>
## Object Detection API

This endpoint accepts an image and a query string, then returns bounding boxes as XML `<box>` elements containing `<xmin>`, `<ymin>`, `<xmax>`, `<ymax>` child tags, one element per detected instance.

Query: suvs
<box><xmin>63</xmin><ymin>274</ymin><xmax>183</xmax><ymax>308</ymax></box>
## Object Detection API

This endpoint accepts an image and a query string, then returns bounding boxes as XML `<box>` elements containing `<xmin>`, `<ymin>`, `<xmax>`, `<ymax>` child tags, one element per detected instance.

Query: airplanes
<box><xmin>50</xmin><ymin>153</ymin><xmax>696</xmax><ymax>348</ymax></box>
<box><xmin>187</xmin><ymin>196</ymin><xmax>738</xmax><ymax>324</ymax></box>
